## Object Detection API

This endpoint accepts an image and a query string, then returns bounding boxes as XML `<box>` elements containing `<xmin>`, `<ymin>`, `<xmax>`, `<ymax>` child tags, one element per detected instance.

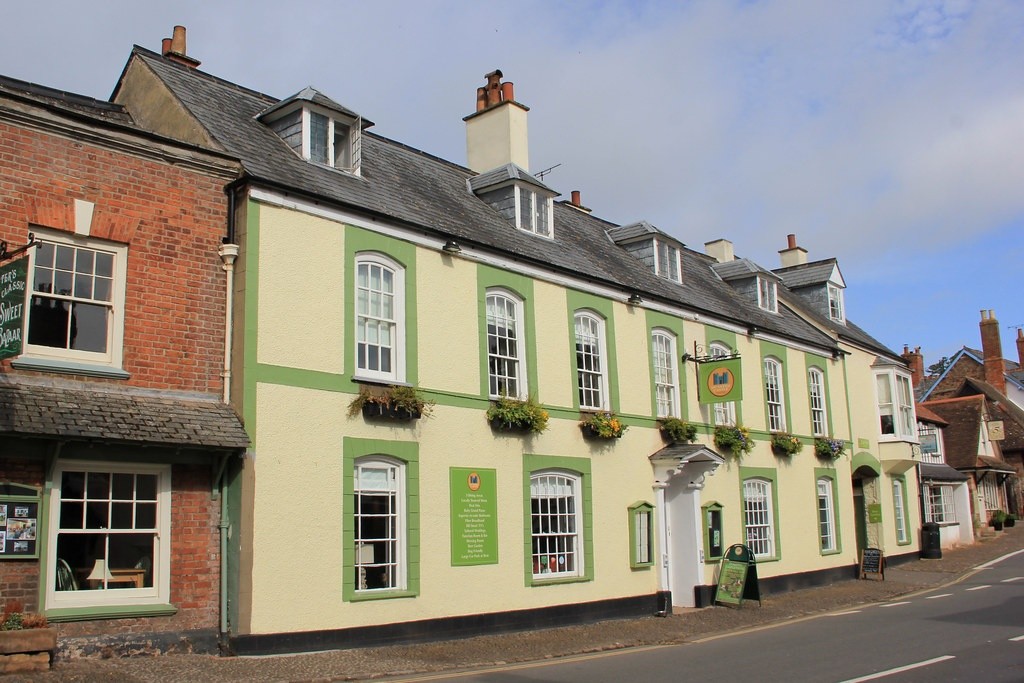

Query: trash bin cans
<box><xmin>921</xmin><ymin>522</ymin><xmax>942</xmax><ymax>559</ymax></box>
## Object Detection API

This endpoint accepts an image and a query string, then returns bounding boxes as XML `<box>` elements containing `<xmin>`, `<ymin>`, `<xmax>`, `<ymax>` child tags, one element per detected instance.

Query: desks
<box><xmin>76</xmin><ymin>566</ymin><xmax>146</xmax><ymax>589</ymax></box>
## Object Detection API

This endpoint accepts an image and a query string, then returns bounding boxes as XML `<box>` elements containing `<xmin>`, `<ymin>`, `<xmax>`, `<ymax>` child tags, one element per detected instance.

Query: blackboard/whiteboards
<box><xmin>860</xmin><ymin>548</ymin><xmax>884</xmax><ymax>575</ymax></box>
<box><xmin>0</xmin><ymin>495</ymin><xmax>41</xmax><ymax>559</ymax></box>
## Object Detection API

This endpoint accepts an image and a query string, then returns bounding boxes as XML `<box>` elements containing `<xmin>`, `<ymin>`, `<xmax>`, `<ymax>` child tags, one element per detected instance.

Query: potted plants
<box><xmin>992</xmin><ymin>511</ymin><xmax>1018</xmax><ymax>531</ymax></box>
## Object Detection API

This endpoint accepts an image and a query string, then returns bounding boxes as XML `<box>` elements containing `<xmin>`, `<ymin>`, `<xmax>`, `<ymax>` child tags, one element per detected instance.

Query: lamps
<box><xmin>747</xmin><ymin>324</ymin><xmax>760</xmax><ymax>336</ymax></box>
<box><xmin>627</xmin><ymin>290</ymin><xmax>644</xmax><ymax>306</ymax></box>
<box><xmin>85</xmin><ymin>559</ymin><xmax>114</xmax><ymax>589</ymax></box>
<box><xmin>442</xmin><ymin>241</ymin><xmax>463</xmax><ymax>254</ymax></box>
<box><xmin>355</xmin><ymin>542</ymin><xmax>375</xmax><ymax>589</ymax></box>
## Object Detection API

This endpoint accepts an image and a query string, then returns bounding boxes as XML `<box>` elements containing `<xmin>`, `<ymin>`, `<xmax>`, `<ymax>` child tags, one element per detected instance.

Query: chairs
<box><xmin>56</xmin><ymin>558</ymin><xmax>78</xmax><ymax>590</ymax></box>
